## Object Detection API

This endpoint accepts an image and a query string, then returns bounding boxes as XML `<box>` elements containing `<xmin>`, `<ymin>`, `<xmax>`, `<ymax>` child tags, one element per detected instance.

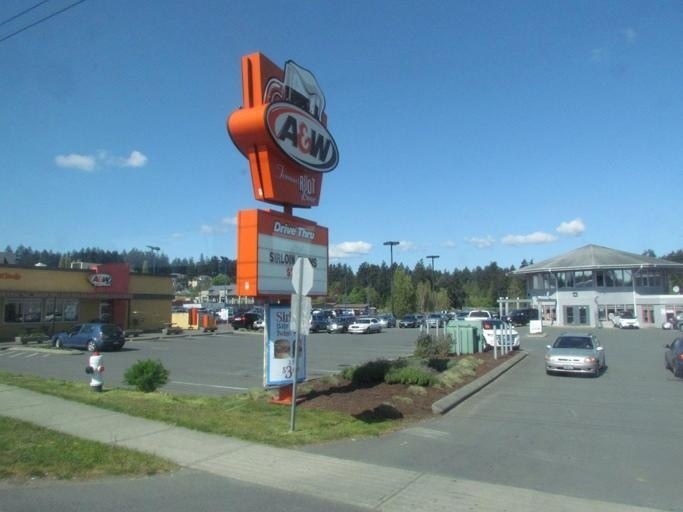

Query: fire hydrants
<box><xmin>85</xmin><ymin>350</ymin><xmax>105</xmax><ymax>393</ymax></box>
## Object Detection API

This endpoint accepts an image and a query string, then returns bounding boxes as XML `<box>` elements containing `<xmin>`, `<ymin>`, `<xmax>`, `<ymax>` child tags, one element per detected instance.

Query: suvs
<box><xmin>482</xmin><ymin>317</ymin><xmax>521</xmax><ymax>353</ymax></box>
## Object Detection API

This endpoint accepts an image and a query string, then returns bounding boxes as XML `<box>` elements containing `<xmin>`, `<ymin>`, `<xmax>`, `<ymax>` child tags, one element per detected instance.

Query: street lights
<box><xmin>424</xmin><ymin>255</ymin><xmax>439</xmax><ymax>300</ymax></box>
<box><xmin>145</xmin><ymin>245</ymin><xmax>160</xmax><ymax>273</ymax></box>
<box><xmin>382</xmin><ymin>241</ymin><xmax>399</xmax><ymax>315</ymax></box>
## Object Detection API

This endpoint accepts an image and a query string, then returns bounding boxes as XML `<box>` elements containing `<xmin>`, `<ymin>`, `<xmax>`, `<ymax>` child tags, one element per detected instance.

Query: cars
<box><xmin>49</xmin><ymin>320</ymin><xmax>124</xmax><ymax>353</ymax></box>
<box><xmin>662</xmin><ymin>312</ymin><xmax>683</xmax><ymax>331</ymax></box>
<box><xmin>613</xmin><ymin>311</ymin><xmax>639</xmax><ymax>330</ymax></box>
<box><xmin>543</xmin><ymin>332</ymin><xmax>605</xmax><ymax>378</ymax></box>
<box><xmin>662</xmin><ymin>336</ymin><xmax>683</xmax><ymax>378</ymax></box>
